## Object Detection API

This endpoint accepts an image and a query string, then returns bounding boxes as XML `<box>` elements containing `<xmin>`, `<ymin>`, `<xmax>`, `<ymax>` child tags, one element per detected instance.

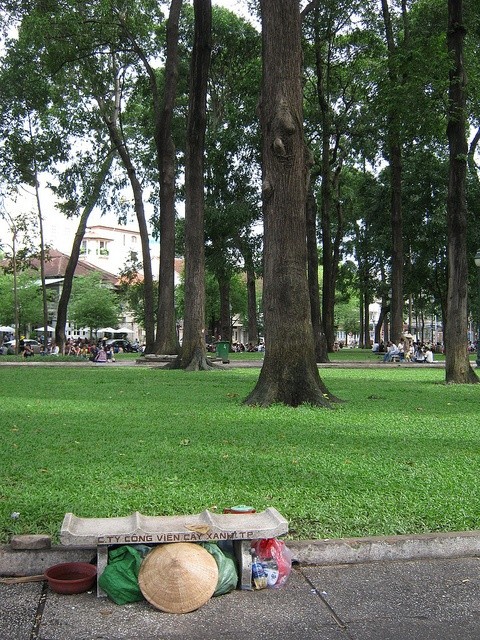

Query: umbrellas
<box><xmin>0</xmin><ymin>325</ymin><xmax>16</xmax><ymax>333</ymax></box>
<box><xmin>97</xmin><ymin>327</ymin><xmax>118</xmax><ymax>332</ymax></box>
<box><xmin>35</xmin><ymin>325</ymin><xmax>56</xmax><ymax>332</ymax></box>
<box><xmin>83</xmin><ymin>327</ymin><xmax>96</xmax><ymax>332</ymax></box>
<box><xmin>117</xmin><ymin>327</ymin><xmax>134</xmax><ymax>333</ymax></box>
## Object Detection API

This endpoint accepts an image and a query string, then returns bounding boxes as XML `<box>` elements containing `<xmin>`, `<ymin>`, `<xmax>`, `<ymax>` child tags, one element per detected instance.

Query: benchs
<box><xmin>59</xmin><ymin>506</ymin><xmax>290</xmax><ymax>599</ymax></box>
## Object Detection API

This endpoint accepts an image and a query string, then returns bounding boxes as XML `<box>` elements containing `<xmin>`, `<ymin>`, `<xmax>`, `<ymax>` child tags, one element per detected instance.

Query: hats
<box><xmin>138</xmin><ymin>543</ymin><xmax>219</xmax><ymax>614</ymax></box>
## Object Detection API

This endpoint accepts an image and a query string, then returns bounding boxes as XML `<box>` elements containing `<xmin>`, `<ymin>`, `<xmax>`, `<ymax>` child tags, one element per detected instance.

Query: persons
<box><xmin>333</xmin><ymin>333</ymin><xmax>358</xmax><ymax>351</ymax></box>
<box><xmin>230</xmin><ymin>343</ymin><xmax>265</xmax><ymax>352</ymax></box>
<box><xmin>206</xmin><ymin>343</ymin><xmax>216</xmax><ymax>352</ymax></box>
<box><xmin>0</xmin><ymin>332</ymin><xmax>146</xmax><ymax>363</ymax></box>
<box><xmin>371</xmin><ymin>338</ymin><xmax>443</xmax><ymax>362</ymax></box>
<box><xmin>467</xmin><ymin>339</ymin><xmax>477</xmax><ymax>353</ymax></box>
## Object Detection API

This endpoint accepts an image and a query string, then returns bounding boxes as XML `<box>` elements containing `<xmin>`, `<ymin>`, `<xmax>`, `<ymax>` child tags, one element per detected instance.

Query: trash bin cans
<box><xmin>3</xmin><ymin>343</ymin><xmax>12</xmax><ymax>355</ymax></box>
<box><xmin>214</xmin><ymin>340</ymin><xmax>230</xmax><ymax>364</ymax></box>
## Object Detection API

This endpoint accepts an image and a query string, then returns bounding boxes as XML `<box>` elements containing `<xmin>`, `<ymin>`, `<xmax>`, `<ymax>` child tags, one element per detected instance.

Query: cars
<box><xmin>105</xmin><ymin>339</ymin><xmax>129</xmax><ymax>350</ymax></box>
<box><xmin>3</xmin><ymin>339</ymin><xmax>44</xmax><ymax>354</ymax></box>
